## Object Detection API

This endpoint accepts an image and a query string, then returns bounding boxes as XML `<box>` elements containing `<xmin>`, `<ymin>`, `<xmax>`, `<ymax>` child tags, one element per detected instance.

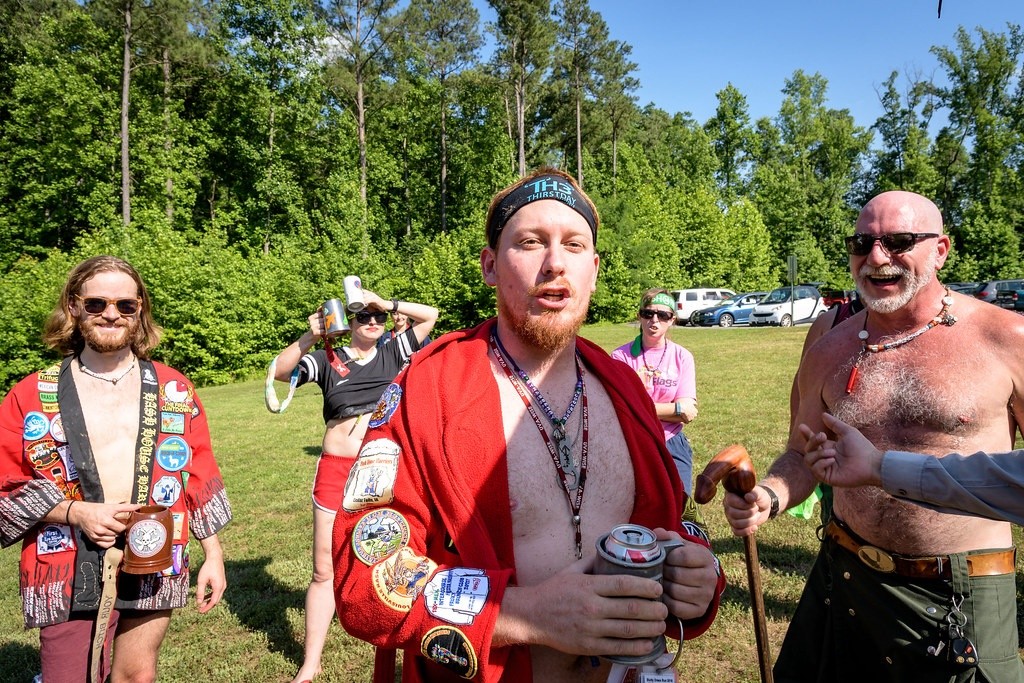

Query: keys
<box><xmin>948</xmin><ymin>594</ymin><xmax>980</xmax><ymax>668</ymax></box>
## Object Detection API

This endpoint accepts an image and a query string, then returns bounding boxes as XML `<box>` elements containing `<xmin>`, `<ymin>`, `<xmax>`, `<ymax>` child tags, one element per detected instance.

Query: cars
<box><xmin>972</xmin><ymin>278</ymin><xmax>1024</xmax><ymax>304</ymax></box>
<box><xmin>748</xmin><ymin>281</ymin><xmax>830</xmax><ymax>328</ymax></box>
<box><xmin>941</xmin><ymin>283</ymin><xmax>976</xmax><ymax>298</ymax></box>
<box><xmin>695</xmin><ymin>293</ymin><xmax>769</xmax><ymax>327</ymax></box>
<box><xmin>992</xmin><ymin>289</ymin><xmax>1024</xmax><ymax>310</ymax></box>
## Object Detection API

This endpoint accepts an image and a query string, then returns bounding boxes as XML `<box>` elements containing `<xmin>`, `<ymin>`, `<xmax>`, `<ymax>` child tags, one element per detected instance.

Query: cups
<box><xmin>112</xmin><ymin>504</ymin><xmax>174</xmax><ymax>575</ymax></box>
<box><xmin>316</xmin><ymin>298</ymin><xmax>351</xmax><ymax>337</ymax></box>
<box><xmin>592</xmin><ymin>531</ymin><xmax>685</xmax><ymax>667</ymax></box>
<box><xmin>342</xmin><ymin>275</ymin><xmax>369</xmax><ymax>312</ymax></box>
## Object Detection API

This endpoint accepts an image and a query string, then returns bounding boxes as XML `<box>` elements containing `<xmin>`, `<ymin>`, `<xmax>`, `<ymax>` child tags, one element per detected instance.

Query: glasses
<box><xmin>74</xmin><ymin>293</ymin><xmax>143</xmax><ymax>316</ymax></box>
<box><xmin>845</xmin><ymin>232</ymin><xmax>939</xmax><ymax>256</ymax></box>
<box><xmin>347</xmin><ymin>310</ymin><xmax>388</xmax><ymax>324</ymax></box>
<box><xmin>639</xmin><ymin>309</ymin><xmax>673</xmax><ymax>321</ymax></box>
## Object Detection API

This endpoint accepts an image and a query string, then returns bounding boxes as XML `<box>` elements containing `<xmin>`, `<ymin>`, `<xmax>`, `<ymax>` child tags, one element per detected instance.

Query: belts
<box><xmin>825</xmin><ymin>521</ymin><xmax>1015</xmax><ymax>579</ymax></box>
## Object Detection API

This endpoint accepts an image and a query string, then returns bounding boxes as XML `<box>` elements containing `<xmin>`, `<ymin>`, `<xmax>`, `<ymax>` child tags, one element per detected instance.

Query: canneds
<box><xmin>605</xmin><ymin>523</ymin><xmax>660</xmax><ymax>563</ymax></box>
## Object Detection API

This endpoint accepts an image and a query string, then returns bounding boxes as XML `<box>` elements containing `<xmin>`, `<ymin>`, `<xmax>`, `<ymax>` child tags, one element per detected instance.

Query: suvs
<box><xmin>820</xmin><ymin>289</ymin><xmax>857</xmax><ymax>310</ymax></box>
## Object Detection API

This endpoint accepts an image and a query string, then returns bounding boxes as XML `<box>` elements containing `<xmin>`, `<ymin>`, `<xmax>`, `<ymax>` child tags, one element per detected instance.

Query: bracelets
<box><xmin>756</xmin><ymin>484</ymin><xmax>780</xmax><ymax>520</ymax></box>
<box><xmin>675</xmin><ymin>401</ymin><xmax>682</xmax><ymax>416</ymax></box>
<box><xmin>66</xmin><ymin>500</ymin><xmax>76</xmax><ymax>525</ymax></box>
<box><xmin>390</xmin><ymin>299</ymin><xmax>399</xmax><ymax>313</ymax></box>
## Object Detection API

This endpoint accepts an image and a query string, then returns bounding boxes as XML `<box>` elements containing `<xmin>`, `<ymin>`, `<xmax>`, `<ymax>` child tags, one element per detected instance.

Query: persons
<box><xmin>265</xmin><ymin>275</ymin><xmax>438</xmax><ymax>683</ymax></box>
<box><xmin>607</xmin><ymin>289</ymin><xmax>698</xmax><ymax>519</ymax></box>
<box><xmin>377</xmin><ymin>311</ymin><xmax>430</xmax><ymax>348</ymax></box>
<box><xmin>724</xmin><ymin>189</ymin><xmax>1024</xmax><ymax>683</ymax></box>
<box><xmin>0</xmin><ymin>255</ymin><xmax>232</xmax><ymax>683</ymax></box>
<box><xmin>328</xmin><ymin>168</ymin><xmax>724</xmax><ymax>683</ymax></box>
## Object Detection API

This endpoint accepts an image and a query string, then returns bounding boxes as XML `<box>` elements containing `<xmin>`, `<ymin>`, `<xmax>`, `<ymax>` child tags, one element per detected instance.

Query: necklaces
<box><xmin>74</xmin><ymin>355</ymin><xmax>136</xmax><ymax>385</ymax></box>
<box><xmin>641</xmin><ymin>334</ymin><xmax>667</xmax><ymax>376</ymax></box>
<box><xmin>494</xmin><ymin>326</ymin><xmax>589</xmax><ymax>561</ymax></box>
<box><xmin>846</xmin><ymin>285</ymin><xmax>959</xmax><ymax>394</ymax></box>
<box><xmin>352</xmin><ymin>339</ymin><xmax>375</xmax><ymax>360</ymax></box>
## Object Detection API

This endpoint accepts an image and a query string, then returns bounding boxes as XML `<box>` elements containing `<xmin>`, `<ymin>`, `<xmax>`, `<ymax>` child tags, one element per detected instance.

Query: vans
<box><xmin>670</xmin><ymin>288</ymin><xmax>738</xmax><ymax>328</ymax></box>
